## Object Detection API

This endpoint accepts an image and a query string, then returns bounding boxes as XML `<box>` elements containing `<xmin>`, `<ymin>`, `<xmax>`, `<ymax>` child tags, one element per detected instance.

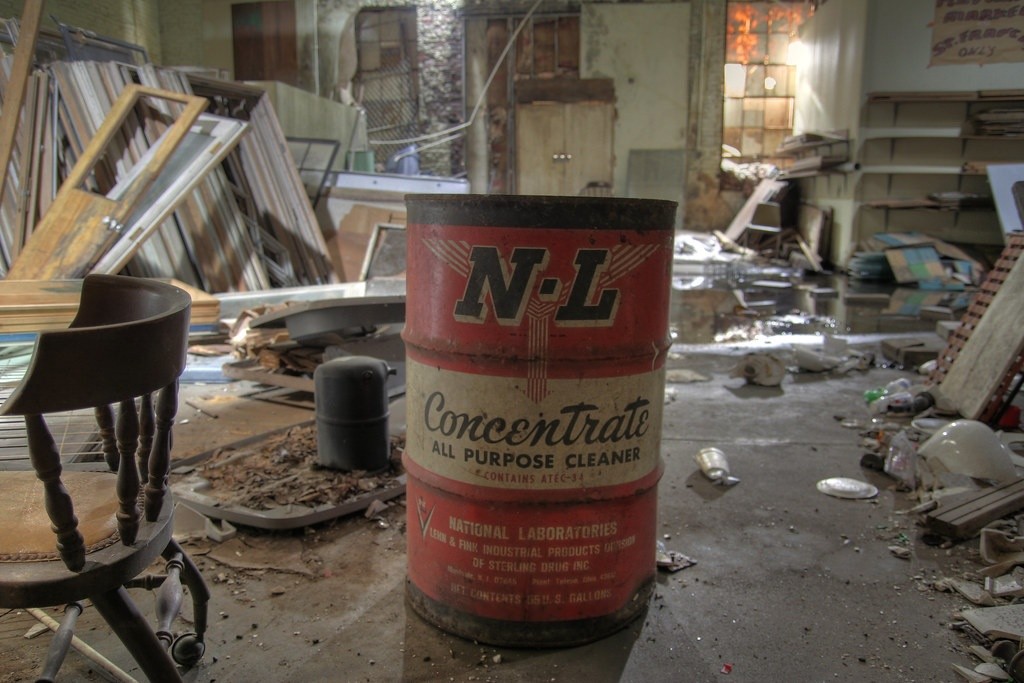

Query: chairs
<box><xmin>0</xmin><ymin>272</ymin><xmax>209</xmax><ymax>682</ymax></box>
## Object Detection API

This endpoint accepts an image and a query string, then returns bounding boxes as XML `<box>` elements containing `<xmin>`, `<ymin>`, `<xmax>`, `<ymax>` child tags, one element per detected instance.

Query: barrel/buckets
<box><xmin>401</xmin><ymin>194</ymin><xmax>681</xmax><ymax>648</ymax></box>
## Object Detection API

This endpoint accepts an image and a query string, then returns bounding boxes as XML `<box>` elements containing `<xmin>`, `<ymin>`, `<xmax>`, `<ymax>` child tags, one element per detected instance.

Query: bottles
<box><xmin>864</xmin><ymin>378</ymin><xmax>941</xmax><ymax>414</ymax></box>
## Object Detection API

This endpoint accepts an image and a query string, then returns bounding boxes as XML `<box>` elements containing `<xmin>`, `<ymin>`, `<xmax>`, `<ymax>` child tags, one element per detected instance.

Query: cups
<box><xmin>694</xmin><ymin>447</ymin><xmax>731</xmax><ymax>480</ymax></box>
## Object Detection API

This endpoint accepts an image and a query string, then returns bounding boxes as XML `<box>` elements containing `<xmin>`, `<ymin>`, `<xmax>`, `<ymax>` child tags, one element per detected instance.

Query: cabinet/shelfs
<box><xmin>518</xmin><ymin>104</ymin><xmax>616</xmax><ymax>200</ymax></box>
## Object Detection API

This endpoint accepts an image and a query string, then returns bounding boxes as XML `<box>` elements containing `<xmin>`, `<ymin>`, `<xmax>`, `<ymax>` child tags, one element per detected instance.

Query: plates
<box><xmin>816</xmin><ymin>478</ymin><xmax>878</xmax><ymax>499</ymax></box>
